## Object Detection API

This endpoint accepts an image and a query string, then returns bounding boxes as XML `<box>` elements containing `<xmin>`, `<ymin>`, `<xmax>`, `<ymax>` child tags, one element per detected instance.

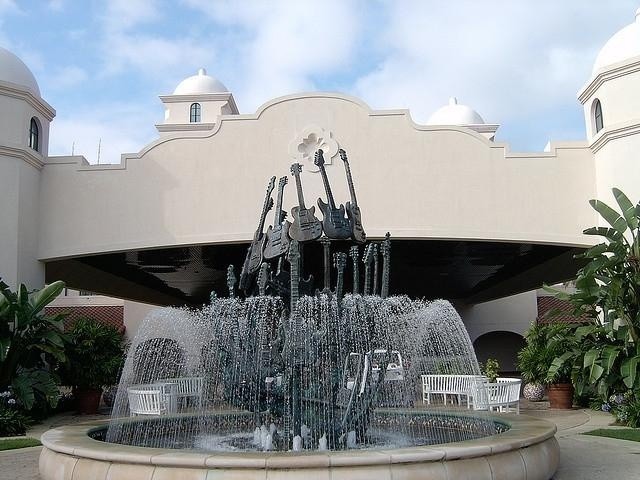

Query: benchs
<box><xmin>153</xmin><ymin>377</ymin><xmax>205</xmax><ymax>407</ymax></box>
<box><xmin>127</xmin><ymin>382</ymin><xmax>179</xmax><ymax>416</ymax></box>
<box><xmin>472</xmin><ymin>377</ymin><xmax>522</xmax><ymax>415</ymax></box>
<box><xmin>422</xmin><ymin>374</ymin><xmax>487</xmax><ymax>403</ymax></box>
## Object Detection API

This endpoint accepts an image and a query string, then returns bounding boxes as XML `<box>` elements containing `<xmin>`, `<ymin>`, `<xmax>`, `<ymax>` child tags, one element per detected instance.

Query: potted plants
<box><xmin>519</xmin><ymin>319</ymin><xmax>580</xmax><ymax>410</ymax></box>
<box><xmin>518</xmin><ymin>348</ymin><xmax>545</xmax><ymax>402</ymax></box>
